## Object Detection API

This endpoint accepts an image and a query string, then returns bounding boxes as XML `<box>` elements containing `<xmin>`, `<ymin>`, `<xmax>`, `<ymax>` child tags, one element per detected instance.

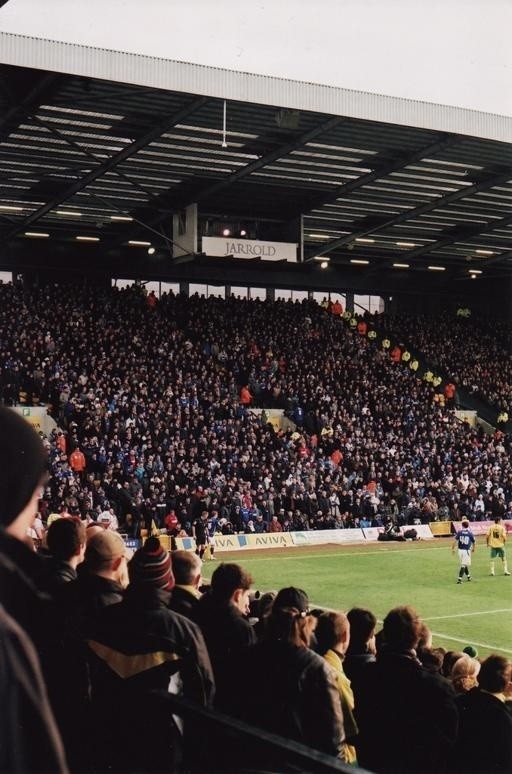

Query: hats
<box><xmin>274</xmin><ymin>587</ymin><xmax>310</xmax><ymax>617</ymax></box>
<box><xmin>1</xmin><ymin>405</ymin><xmax>51</xmax><ymax>524</ymax></box>
<box><xmin>132</xmin><ymin>536</ymin><xmax>176</xmax><ymax>590</ymax></box>
<box><xmin>463</xmin><ymin>646</ymin><xmax>479</xmax><ymax>659</ymax></box>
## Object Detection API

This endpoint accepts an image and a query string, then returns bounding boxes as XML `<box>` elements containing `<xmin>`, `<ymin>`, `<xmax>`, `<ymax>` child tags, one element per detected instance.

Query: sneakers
<box><xmin>504</xmin><ymin>571</ymin><xmax>511</xmax><ymax>575</ymax></box>
<box><xmin>457</xmin><ymin>578</ymin><xmax>463</xmax><ymax>584</ymax></box>
<box><xmin>468</xmin><ymin>575</ymin><xmax>472</xmax><ymax>581</ymax></box>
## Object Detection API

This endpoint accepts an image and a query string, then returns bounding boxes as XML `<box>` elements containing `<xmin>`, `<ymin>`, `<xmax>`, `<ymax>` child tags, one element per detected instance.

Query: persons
<box><xmin>1</xmin><ymin>274</ymin><xmax>512</xmax><ymax>773</ymax></box>
